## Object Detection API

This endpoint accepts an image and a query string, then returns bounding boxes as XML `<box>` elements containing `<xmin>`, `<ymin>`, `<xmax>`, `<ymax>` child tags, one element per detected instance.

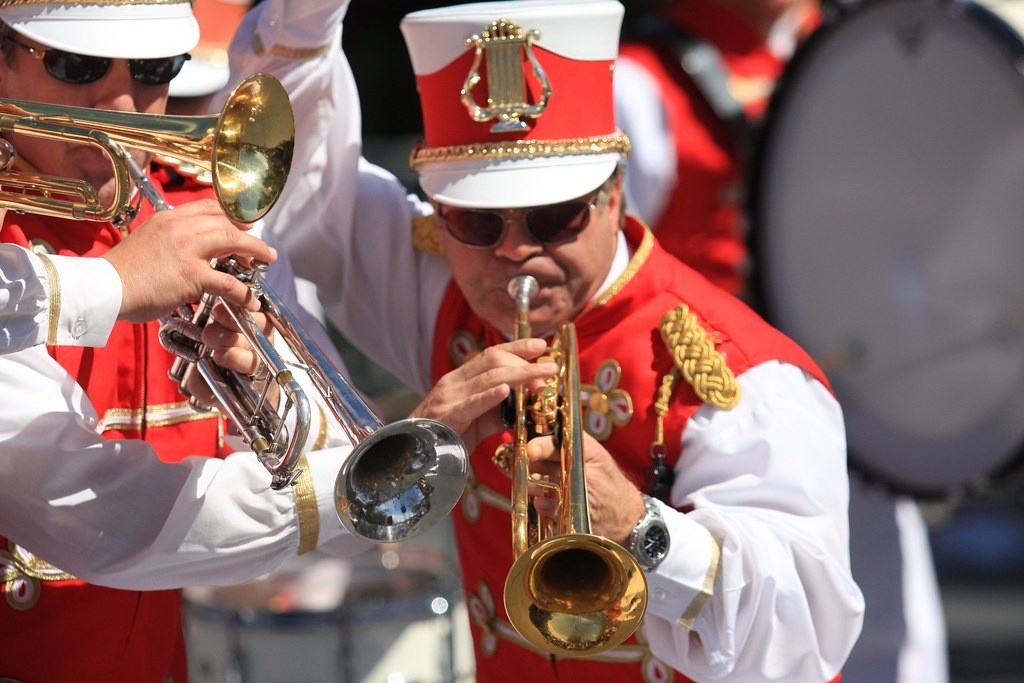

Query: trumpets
<box><xmin>488</xmin><ymin>273</ymin><xmax>653</xmax><ymax>662</ymax></box>
<box><xmin>0</xmin><ymin>68</ymin><xmax>299</xmax><ymax>230</ymax></box>
<box><xmin>74</xmin><ymin>123</ymin><xmax>478</xmax><ymax>548</ymax></box>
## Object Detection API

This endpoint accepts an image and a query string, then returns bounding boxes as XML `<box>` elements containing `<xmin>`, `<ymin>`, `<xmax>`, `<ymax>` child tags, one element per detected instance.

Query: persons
<box><xmin>0</xmin><ymin>0</ymin><xmax>950</xmax><ymax>683</ymax></box>
<box><xmin>205</xmin><ymin>0</ymin><xmax>865</xmax><ymax>683</ymax></box>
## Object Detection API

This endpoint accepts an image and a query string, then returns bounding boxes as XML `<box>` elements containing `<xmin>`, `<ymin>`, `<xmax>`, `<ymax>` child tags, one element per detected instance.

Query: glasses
<box><xmin>6</xmin><ymin>36</ymin><xmax>192</xmax><ymax>85</ymax></box>
<box><xmin>438</xmin><ymin>194</ymin><xmax>599</xmax><ymax>249</ymax></box>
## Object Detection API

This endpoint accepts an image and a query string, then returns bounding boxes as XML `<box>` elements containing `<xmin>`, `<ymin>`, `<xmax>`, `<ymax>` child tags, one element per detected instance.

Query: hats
<box><xmin>398</xmin><ymin>0</ymin><xmax>633</xmax><ymax>209</ymax></box>
<box><xmin>0</xmin><ymin>1</ymin><xmax>201</xmax><ymax>60</ymax></box>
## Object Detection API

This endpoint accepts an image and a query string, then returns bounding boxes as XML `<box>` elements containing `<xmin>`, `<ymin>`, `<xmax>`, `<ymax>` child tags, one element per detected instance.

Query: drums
<box><xmin>733</xmin><ymin>4</ymin><xmax>1022</xmax><ymax>509</ymax></box>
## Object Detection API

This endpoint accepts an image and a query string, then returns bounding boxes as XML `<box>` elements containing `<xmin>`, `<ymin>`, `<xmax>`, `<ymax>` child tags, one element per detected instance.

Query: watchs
<box><xmin>630</xmin><ymin>493</ymin><xmax>671</xmax><ymax>572</ymax></box>
